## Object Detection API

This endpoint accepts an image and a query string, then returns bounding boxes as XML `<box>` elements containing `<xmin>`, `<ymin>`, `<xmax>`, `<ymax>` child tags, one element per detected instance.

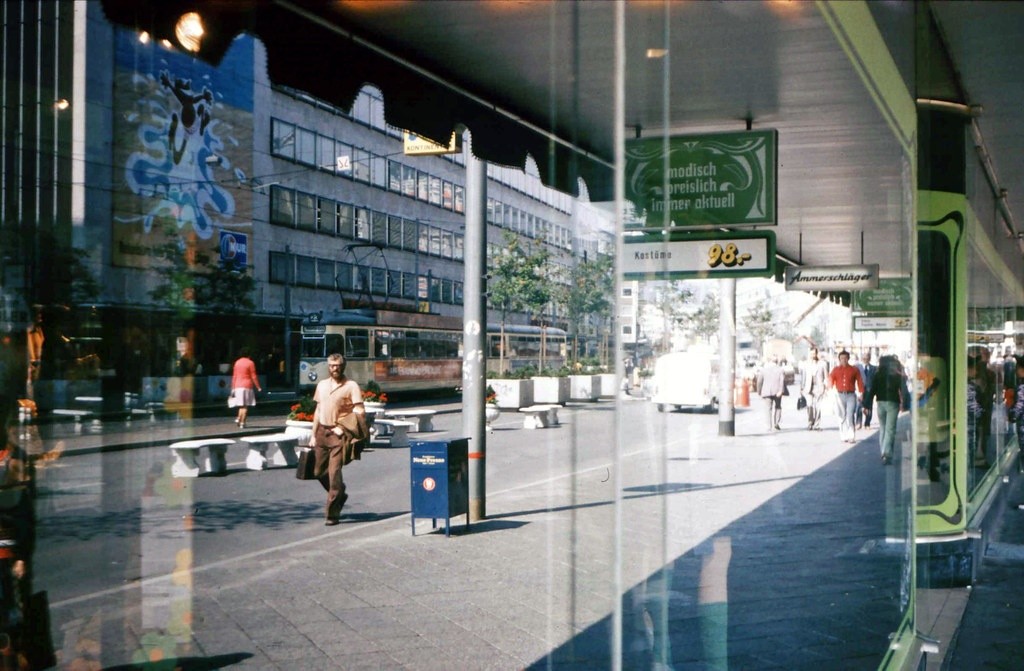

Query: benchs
<box><xmin>167</xmin><ymin>438</ymin><xmax>237</xmax><ymax>478</ymax></box>
<box><xmin>372</xmin><ymin>419</ymin><xmax>415</xmax><ymax>449</ymax></box>
<box><xmin>386</xmin><ymin>409</ymin><xmax>437</xmax><ymax>432</ymax></box>
<box><xmin>519</xmin><ymin>404</ymin><xmax>563</xmax><ymax>429</ymax></box>
<box><xmin>240</xmin><ymin>433</ymin><xmax>302</xmax><ymax>471</ymax></box>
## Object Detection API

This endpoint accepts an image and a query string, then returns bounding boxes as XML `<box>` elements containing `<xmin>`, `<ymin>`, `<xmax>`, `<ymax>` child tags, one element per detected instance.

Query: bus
<box><xmin>294</xmin><ymin>241</ymin><xmax>571</xmax><ymax>403</ymax></box>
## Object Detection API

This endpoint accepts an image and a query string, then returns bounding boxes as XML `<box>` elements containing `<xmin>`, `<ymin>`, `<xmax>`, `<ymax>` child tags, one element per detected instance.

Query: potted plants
<box><xmin>486</xmin><ymin>357</ymin><xmax>652</xmax><ymax>413</ymax></box>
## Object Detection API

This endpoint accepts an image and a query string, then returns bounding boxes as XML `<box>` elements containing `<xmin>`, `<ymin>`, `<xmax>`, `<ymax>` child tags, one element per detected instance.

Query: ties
<box><xmin>864</xmin><ymin>364</ymin><xmax>868</xmax><ymax>376</ymax></box>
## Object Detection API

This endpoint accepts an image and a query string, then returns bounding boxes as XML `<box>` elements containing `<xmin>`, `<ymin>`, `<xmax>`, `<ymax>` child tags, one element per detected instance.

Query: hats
<box><xmin>1012</xmin><ymin>355</ymin><xmax>1024</xmax><ymax>367</ymax></box>
<box><xmin>968</xmin><ymin>354</ymin><xmax>981</xmax><ymax>364</ymax></box>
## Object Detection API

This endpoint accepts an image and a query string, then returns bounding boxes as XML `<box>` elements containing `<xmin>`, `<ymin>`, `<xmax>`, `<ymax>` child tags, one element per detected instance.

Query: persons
<box><xmin>308</xmin><ymin>353</ymin><xmax>365</xmax><ymax>526</ymax></box>
<box><xmin>228</xmin><ymin>347</ymin><xmax>261</xmax><ymax>429</ymax></box>
<box><xmin>0</xmin><ymin>426</ymin><xmax>37</xmax><ymax>671</ymax></box>
<box><xmin>757</xmin><ymin>356</ymin><xmax>785</xmax><ymax>431</ymax></box>
<box><xmin>799</xmin><ymin>348</ymin><xmax>829</xmax><ymax>430</ymax></box>
<box><xmin>862</xmin><ymin>356</ymin><xmax>908</xmax><ymax>465</ymax></box>
<box><xmin>825</xmin><ymin>350</ymin><xmax>865</xmax><ymax>443</ymax></box>
<box><xmin>1006</xmin><ymin>356</ymin><xmax>1024</xmax><ymax>510</ymax></box>
<box><xmin>967</xmin><ymin>354</ymin><xmax>1018</xmax><ymax>498</ymax></box>
<box><xmin>852</xmin><ymin>352</ymin><xmax>878</xmax><ymax>429</ymax></box>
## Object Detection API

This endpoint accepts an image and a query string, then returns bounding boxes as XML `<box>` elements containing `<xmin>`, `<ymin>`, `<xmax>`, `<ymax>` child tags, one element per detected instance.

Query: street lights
<box><xmin>735</xmin><ymin>379</ymin><xmax>751</xmax><ymax>408</ymax></box>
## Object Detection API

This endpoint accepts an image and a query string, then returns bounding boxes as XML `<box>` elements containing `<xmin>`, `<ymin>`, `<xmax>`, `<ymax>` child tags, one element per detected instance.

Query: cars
<box><xmin>648</xmin><ymin>343</ymin><xmax>795</xmax><ymax>415</ymax></box>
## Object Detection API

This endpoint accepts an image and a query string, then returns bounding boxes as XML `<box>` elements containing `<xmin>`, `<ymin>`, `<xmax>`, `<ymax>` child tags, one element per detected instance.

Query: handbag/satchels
<box><xmin>797</xmin><ymin>392</ymin><xmax>807</xmax><ymax>410</ymax></box>
<box><xmin>227</xmin><ymin>395</ymin><xmax>236</xmax><ymax>408</ymax></box>
<box><xmin>296</xmin><ymin>446</ymin><xmax>318</xmax><ymax>480</ymax></box>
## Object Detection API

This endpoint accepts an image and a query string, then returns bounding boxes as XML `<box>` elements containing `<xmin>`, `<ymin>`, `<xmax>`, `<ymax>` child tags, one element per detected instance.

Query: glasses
<box><xmin>329</xmin><ymin>364</ymin><xmax>342</xmax><ymax>369</ymax></box>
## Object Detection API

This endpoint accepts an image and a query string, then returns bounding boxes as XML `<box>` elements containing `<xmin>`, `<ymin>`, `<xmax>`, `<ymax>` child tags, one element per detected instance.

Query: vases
<box><xmin>284</xmin><ymin>420</ymin><xmax>313</xmax><ymax>446</ymax></box>
<box><xmin>485</xmin><ymin>404</ymin><xmax>501</xmax><ymax>431</ymax></box>
<box><xmin>353</xmin><ymin>402</ymin><xmax>386</xmax><ymax>419</ymax></box>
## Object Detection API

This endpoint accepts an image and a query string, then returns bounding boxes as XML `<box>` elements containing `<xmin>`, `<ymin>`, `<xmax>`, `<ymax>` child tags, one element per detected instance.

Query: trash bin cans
<box><xmin>408</xmin><ymin>437</ymin><xmax>471</xmax><ymax>537</ymax></box>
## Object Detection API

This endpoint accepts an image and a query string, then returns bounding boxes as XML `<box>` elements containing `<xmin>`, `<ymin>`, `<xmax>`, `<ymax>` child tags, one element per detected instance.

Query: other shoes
<box><xmin>234</xmin><ymin>419</ymin><xmax>239</xmax><ymax>423</ymax></box>
<box><xmin>883</xmin><ymin>457</ymin><xmax>891</xmax><ymax>465</ymax></box>
<box><xmin>324</xmin><ymin>518</ymin><xmax>340</xmax><ymax>525</ymax></box>
<box><xmin>239</xmin><ymin>423</ymin><xmax>245</xmax><ymax>428</ymax></box>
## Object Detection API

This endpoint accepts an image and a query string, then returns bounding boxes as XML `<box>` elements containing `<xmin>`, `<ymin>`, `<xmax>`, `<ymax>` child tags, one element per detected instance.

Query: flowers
<box><xmin>288</xmin><ymin>393</ymin><xmax>318</xmax><ymax>422</ymax></box>
<box><xmin>361</xmin><ymin>378</ymin><xmax>387</xmax><ymax>403</ymax></box>
<box><xmin>484</xmin><ymin>385</ymin><xmax>498</xmax><ymax>405</ymax></box>
